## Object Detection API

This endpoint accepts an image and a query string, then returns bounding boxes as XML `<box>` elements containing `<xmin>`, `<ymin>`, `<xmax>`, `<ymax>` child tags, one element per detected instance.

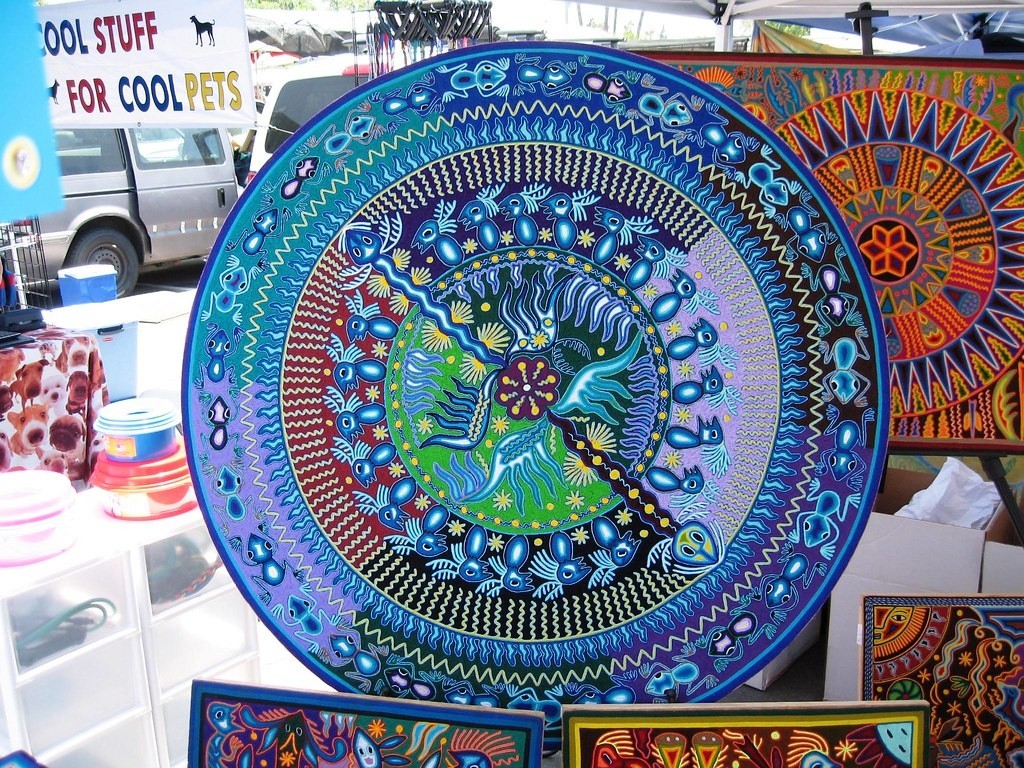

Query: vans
<box><xmin>245</xmin><ymin>51</ymin><xmax>401</xmax><ymax>189</ymax></box>
<box><xmin>0</xmin><ymin>98</ymin><xmax>265</xmax><ymax>302</ymax></box>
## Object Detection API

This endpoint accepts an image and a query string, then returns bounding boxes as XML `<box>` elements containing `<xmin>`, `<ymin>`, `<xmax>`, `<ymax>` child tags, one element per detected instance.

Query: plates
<box><xmin>181</xmin><ymin>42</ymin><xmax>892</xmax><ymax>749</ymax></box>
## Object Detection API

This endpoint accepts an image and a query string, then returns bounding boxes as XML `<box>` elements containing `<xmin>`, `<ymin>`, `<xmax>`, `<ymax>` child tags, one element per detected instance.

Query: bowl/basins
<box><xmin>0</xmin><ymin>470</ymin><xmax>79</xmax><ymax>569</ymax></box>
<box><xmin>93</xmin><ymin>395</ymin><xmax>182</xmax><ymax>462</ymax></box>
<box><xmin>88</xmin><ymin>434</ymin><xmax>199</xmax><ymax>520</ymax></box>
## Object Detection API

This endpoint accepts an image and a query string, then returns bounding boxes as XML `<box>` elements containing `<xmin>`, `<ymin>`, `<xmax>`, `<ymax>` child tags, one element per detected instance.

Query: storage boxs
<box><xmin>58</xmin><ymin>263</ymin><xmax>118</xmax><ymax>307</ymax></box>
<box><xmin>1</xmin><ymin>516</ymin><xmax>266</xmax><ymax>768</ymax></box>
<box><xmin>746</xmin><ymin>468</ymin><xmax>1024</xmax><ymax>701</ymax></box>
<box><xmin>77</xmin><ymin>320</ymin><xmax>139</xmax><ymax>402</ymax></box>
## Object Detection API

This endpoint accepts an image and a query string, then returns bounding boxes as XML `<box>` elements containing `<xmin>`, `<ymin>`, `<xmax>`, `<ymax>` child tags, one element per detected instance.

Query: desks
<box><xmin>0</xmin><ymin>323</ymin><xmax>111</xmax><ymax>486</ymax></box>
